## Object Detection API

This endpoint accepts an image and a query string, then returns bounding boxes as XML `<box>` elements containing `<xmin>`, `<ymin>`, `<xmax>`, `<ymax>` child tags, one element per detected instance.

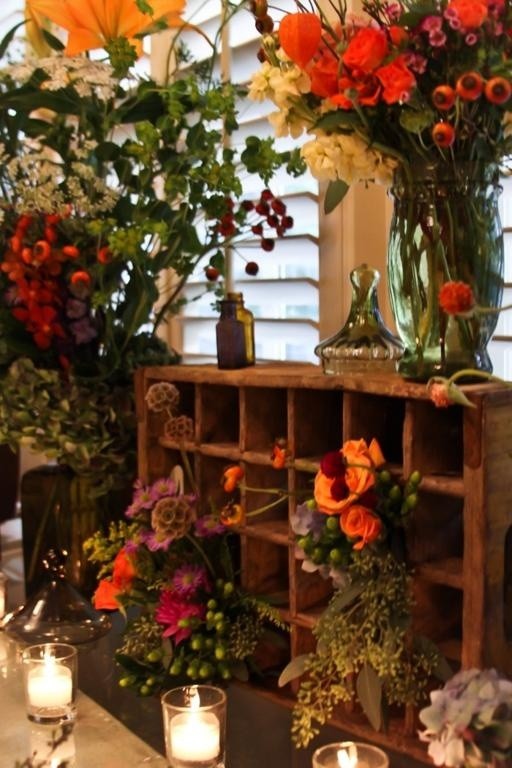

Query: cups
<box><xmin>21</xmin><ymin>641</ymin><xmax>81</xmax><ymax>727</ymax></box>
<box><xmin>308</xmin><ymin>740</ymin><xmax>390</xmax><ymax>768</ymax></box>
<box><xmin>160</xmin><ymin>686</ymin><xmax>226</xmax><ymax>767</ymax></box>
<box><xmin>29</xmin><ymin>722</ymin><xmax>79</xmax><ymax>766</ymax></box>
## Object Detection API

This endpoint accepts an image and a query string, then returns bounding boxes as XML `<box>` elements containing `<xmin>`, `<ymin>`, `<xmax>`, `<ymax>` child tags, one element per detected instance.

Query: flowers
<box><xmin>0</xmin><ymin>1</ymin><xmax>295</xmax><ymax>702</ymax></box>
<box><xmin>292</xmin><ymin>437</ymin><xmax>510</xmax><ymax>768</ymax></box>
<box><xmin>248</xmin><ymin>1</ymin><xmax>512</xmax><ymax>187</ymax></box>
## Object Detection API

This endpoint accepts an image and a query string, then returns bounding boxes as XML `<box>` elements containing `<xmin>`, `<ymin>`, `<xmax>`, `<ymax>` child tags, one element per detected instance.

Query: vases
<box><xmin>388</xmin><ymin>176</ymin><xmax>506</xmax><ymax>386</ymax></box>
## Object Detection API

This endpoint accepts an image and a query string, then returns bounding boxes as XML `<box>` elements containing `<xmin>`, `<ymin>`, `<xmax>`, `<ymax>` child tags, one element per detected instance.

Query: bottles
<box><xmin>313</xmin><ymin>262</ymin><xmax>409</xmax><ymax>376</ymax></box>
<box><xmin>215</xmin><ymin>288</ymin><xmax>258</xmax><ymax>369</ymax></box>
